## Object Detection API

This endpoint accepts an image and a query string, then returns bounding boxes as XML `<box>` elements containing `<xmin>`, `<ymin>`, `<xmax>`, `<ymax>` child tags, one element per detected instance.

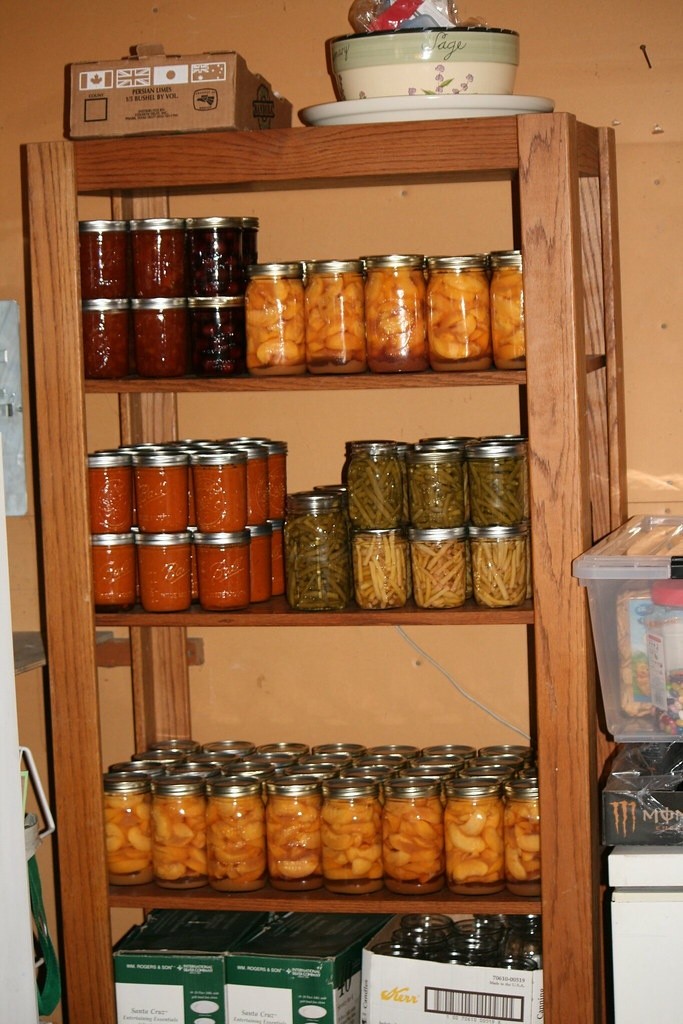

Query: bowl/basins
<box><xmin>328</xmin><ymin>28</ymin><xmax>523</xmax><ymax>102</ymax></box>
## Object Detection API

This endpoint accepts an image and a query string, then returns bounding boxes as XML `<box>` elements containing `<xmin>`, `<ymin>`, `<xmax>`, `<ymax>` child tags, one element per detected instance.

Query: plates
<box><xmin>295</xmin><ymin>96</ymin><xmax>557</xmax><ymax>131</ymax></box>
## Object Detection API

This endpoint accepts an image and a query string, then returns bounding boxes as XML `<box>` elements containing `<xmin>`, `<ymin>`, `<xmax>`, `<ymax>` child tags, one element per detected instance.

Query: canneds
<box><xmin>80</xmin><ymin>217</ymin><xmax>545</xmax><ymax>898</ymax></box>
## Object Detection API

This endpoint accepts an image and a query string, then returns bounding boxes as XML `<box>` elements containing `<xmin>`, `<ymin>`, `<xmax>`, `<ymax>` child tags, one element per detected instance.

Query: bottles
<box><xmin>369</xmin><ymin>912</ymin><xmax>544</xmax><ymax>973</ymax></box>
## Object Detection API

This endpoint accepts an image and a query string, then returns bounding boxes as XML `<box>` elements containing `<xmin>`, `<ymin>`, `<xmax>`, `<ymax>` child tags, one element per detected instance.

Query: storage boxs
<box><xmin>112</xmin><ymin>908</ymin><xmax>545</xmax><ymax>1024</ymax></box>
<box><xmin>601</xmin><ymin>744</ymin><xmax>683</xmax><ymax>847</ymax></box>
<box><xmin>70</xmin><ymin>42</ymin><xmax>294</xmax><ymax>139</ymax></box>
<box><xmin>573</xmin><ymin>513</ymin><xmax>683</xmax><ymax>743</ymax></box>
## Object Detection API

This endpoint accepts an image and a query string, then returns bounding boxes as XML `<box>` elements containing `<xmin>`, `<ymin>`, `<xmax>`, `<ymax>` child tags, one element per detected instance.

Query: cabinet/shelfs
<box><xmin>24</xmin><ymin>112</ymin><xmax>626</xmax><ymax>1024</ymax></box>
